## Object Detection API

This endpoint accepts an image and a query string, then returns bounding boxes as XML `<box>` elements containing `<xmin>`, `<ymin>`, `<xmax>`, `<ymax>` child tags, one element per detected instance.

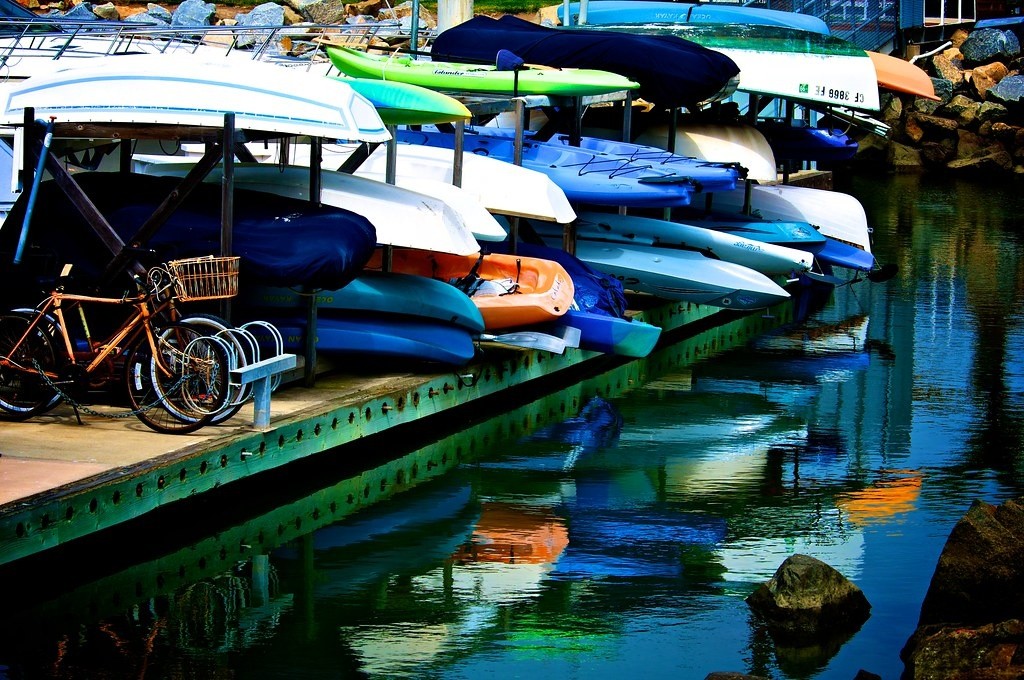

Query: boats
<box><xmin>0</xmin><ymin>1</ymin><xmax>942</xmax><ymax>366</ymax></box>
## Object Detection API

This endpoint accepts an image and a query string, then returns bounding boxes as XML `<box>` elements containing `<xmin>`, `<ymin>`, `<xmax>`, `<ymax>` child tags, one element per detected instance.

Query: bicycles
<box><xmin>0</xmin><ymin>234</ymin><xmax>243</xmax><ymax>435</ymax></box>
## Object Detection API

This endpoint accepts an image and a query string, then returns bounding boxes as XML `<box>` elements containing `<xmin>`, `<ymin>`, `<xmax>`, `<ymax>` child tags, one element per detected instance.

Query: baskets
<box><xmin>168</xmin><ymin>255</ymin><xmax>240</xmax><ymax>303</ymax></box>
